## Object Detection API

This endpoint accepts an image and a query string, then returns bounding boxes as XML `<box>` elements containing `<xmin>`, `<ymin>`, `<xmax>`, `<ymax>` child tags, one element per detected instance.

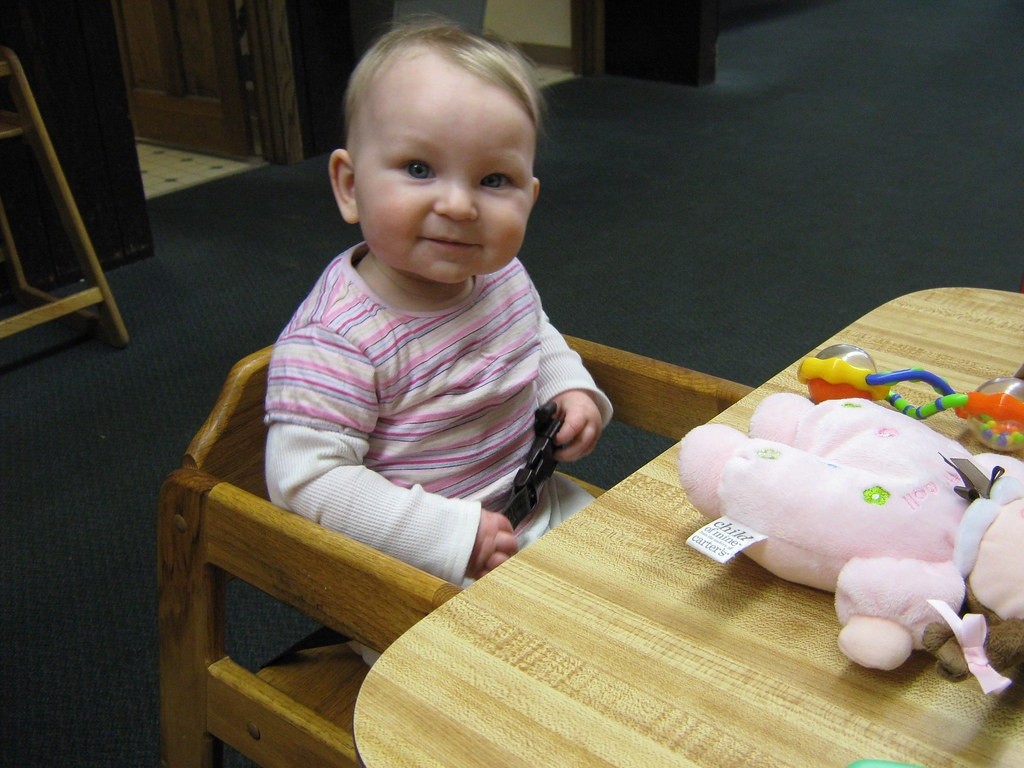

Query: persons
<box><xmin>264</xmin><ymin>15</ymin><xmax>614</xmax><ymax>667</ymax></box>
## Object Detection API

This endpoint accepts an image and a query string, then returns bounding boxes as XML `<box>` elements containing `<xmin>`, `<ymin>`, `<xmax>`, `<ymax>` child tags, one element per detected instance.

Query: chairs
<box><xmin>156</xmin><ymin>327</ymin><xmax>754</xmax><ymax>768</ymax></box>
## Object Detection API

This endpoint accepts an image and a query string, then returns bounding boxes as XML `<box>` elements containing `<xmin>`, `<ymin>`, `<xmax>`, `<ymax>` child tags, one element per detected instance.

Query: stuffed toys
<box><xmin>678</xmin><ymin>392</ymin><xmax>1024</xmax><ymax>696</ymax></box>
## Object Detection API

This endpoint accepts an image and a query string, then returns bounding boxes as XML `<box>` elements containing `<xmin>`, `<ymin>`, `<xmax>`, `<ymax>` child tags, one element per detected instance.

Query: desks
<box><xmin>351</xmin><ymin>286</ymin><xmax>1024</xmax><ymax>768</ymax></box>
